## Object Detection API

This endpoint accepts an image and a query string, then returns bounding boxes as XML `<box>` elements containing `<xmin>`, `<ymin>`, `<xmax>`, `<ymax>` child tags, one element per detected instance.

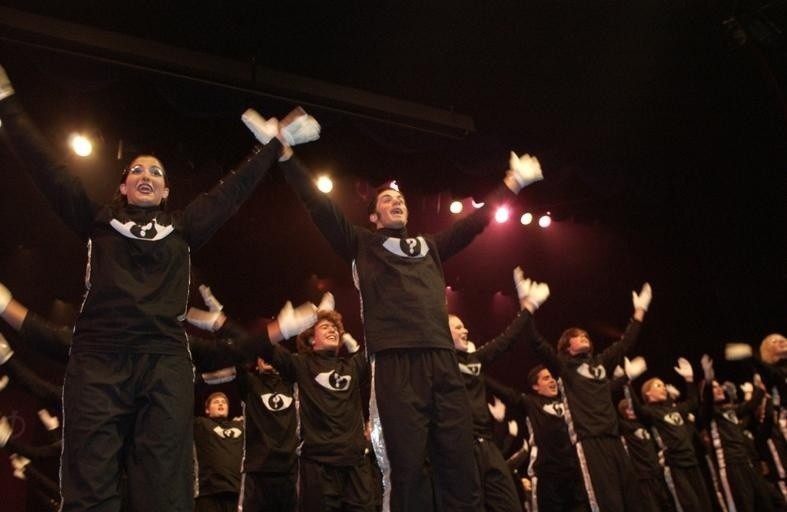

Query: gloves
<box><xmin>241</xmin><ymin>108</ymin><xmax>294</xmax><ymax>163</ymax></box>
<box><xmin>632</xmin><ymin>282</ymin><xmax>652</xmax><ymax>312</ymax></box>
<box><xmin>701</xmin><ymin>343</ymin><xmax>754</xmax><ymax>396</ymax></box>
<box><xmin>280</xmin><ymin>112</ymin><xmax>322</xmax><ymax>146</ymax></box>
<box><xmin>613</xmin><ymin>356</ymin><xmax>681</xmax><ymax>397</ymax></box>
<box><xmin>506</xmin><ymin>150</ymin><xmax>545</xmax><ymax>189</ymax></box>
<box><xmin>342</xmin><ymin>333</ymin><xmax>360</xmax><ymax>354</ymax></box>
<box><xmin>277</xmin><ymin>300</ymin><xmax>318</xmax><ymax>340</ymax></box>
<box><xmin>186</xmin><ymin>284</ymin><xmax>225</xmax><ymax>333</ymax></box>
<box><xmin>674</xmin><ymin>357</ymin><xmax>693</xmax><ymax>377</ymax></box>
<box><xmin>466</xmin><ymin>266</ymin><xmax>550</xmax><ymax>453</ymax></box>
<box><xmin>318</xmin><ymin>291</ymin><xmax>335</xmax><ymax>313</ymax></box>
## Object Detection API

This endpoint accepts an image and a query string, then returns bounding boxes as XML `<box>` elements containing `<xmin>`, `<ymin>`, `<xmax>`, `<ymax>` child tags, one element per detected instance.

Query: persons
<box><xmin>0</xmin><ymin>267</ymin><xmax>787</xmax><ymax>512</ymax></box>
<box><xmin>1</xmin><ymin>69</ymin><xmax>321</xmax><ymax>512</ymax></box>
<box><xmin>242</xmin><ymin>111</ymin><xmax>542</xmax><ymax>512</ymax></box>
